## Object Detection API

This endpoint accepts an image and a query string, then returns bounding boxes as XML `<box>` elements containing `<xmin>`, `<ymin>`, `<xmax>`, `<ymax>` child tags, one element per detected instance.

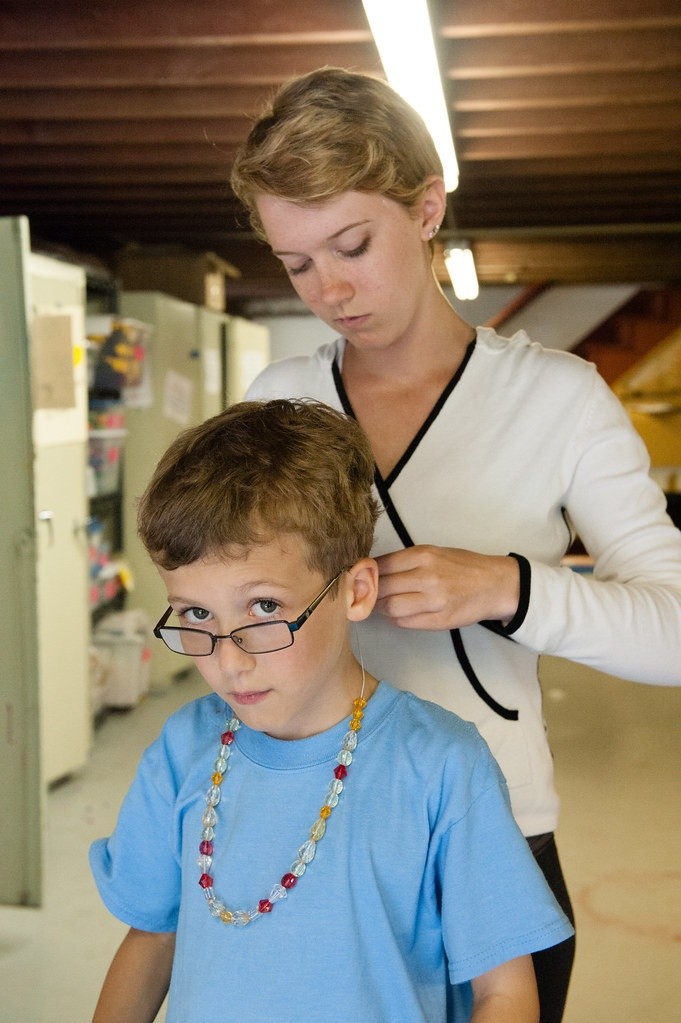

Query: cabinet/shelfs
<box><xmin>80</xmin><ymin>271</ymin><xmax>135</xmax><ymax>719</ymax></box>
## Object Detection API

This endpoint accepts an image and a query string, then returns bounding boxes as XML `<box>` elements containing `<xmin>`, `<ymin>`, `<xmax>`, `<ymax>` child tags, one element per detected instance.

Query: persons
<box><xmin>225</xmin><ymin>66</ymin><xmax>679</xmax><ymax>1022</ymax></box>
<box><xmin>84</xmin><ymin>393</ymin><xmax>584</xmax><ymax>1021</ymax></box>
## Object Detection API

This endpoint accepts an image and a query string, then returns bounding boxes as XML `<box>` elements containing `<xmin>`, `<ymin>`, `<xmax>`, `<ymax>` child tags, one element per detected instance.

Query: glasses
<box><xmin>153</xmin><ymin>563</ymin><xmax>351</xmax><ymax>657</ymax></box>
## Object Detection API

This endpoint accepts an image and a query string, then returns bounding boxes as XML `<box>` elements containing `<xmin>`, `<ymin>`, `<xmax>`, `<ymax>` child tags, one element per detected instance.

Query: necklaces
<box><xmin>198</xmin><ymin>657</ymin><xmax>370</xmax><ymax>928</ymax></box>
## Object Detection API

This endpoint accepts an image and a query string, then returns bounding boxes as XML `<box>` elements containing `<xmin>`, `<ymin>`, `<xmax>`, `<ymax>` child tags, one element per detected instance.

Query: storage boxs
<box><xmin>82</xmin><ymin>303</ymin><xmax>158</xmax><ymax>697</ymax></box>
